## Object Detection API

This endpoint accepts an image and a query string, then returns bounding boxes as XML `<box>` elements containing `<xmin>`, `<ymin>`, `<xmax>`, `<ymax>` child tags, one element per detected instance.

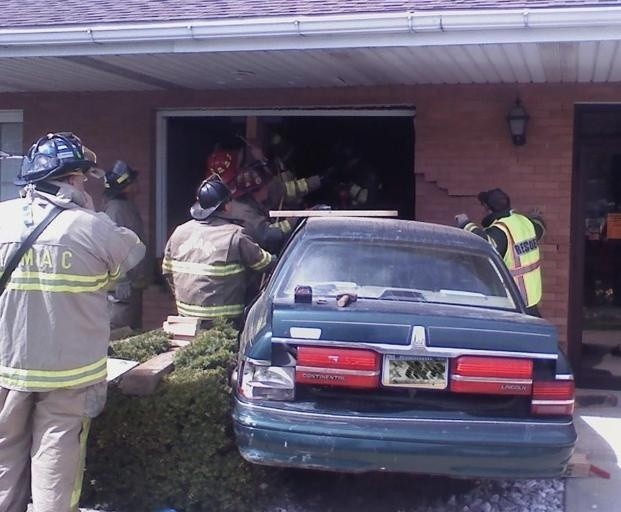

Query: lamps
<box><xmin>506</xmin><ymin>94</ymin><xmax>529</xmax><ymax>147</ymax></box>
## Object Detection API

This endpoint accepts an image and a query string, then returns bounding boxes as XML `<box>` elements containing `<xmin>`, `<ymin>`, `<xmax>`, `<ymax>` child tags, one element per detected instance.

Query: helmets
<box><xmin>235</xmin><ymin>160</ymin><xmax>273</xmax><ymax>192</ymax></box>
<box><xmin>101</xmin><ymin>158</ymin><xmax>141</xmax><ymax>197</ymax></box>
<box><xmin>204</xmin><ymin>148</ymin><xmax>242</xmax><ymax>184</ymax></box>
<box><xmin>13</xmin><ymin>131</ymin><xmax>98</xmax><ymax>187</ymax></box>
<box><xmin>189</xmin><ymin>173</ymin><xmax>233</xmax><ymax>221</ymax></box>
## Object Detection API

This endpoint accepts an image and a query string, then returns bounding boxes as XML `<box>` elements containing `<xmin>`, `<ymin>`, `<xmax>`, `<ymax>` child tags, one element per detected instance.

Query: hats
<box><xmin>477</xmin><ymin>188</ymin><xmax>511</xmax><ymax>212</ymax></box>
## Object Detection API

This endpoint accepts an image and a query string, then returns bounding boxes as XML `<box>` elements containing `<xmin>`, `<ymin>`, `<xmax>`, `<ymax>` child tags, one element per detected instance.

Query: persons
<box><xmin>0</xmin><ymin>127</ymin><xmax>155</xmax><ymax>512</ymax></box>
<box><xmin>455</xmin><ymin>187</ymin><xmax>548</xmax><ymax>319</ymax></box>
<box><xmin>100</xmin><ymin>155</ymin><xmax>149</xmax><ymax>337</ymax></box>
<box><xmin>159</xmin><ymin>130</ymin><xmax>388</xmax><ymax>336</ymax></box>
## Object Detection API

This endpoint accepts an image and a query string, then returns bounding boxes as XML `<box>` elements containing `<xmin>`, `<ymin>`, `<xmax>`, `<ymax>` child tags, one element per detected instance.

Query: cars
<box><xmin>225</xmin><ymin>216</ymin><xmax>578</xmax><ymax>481</ymax></box>
